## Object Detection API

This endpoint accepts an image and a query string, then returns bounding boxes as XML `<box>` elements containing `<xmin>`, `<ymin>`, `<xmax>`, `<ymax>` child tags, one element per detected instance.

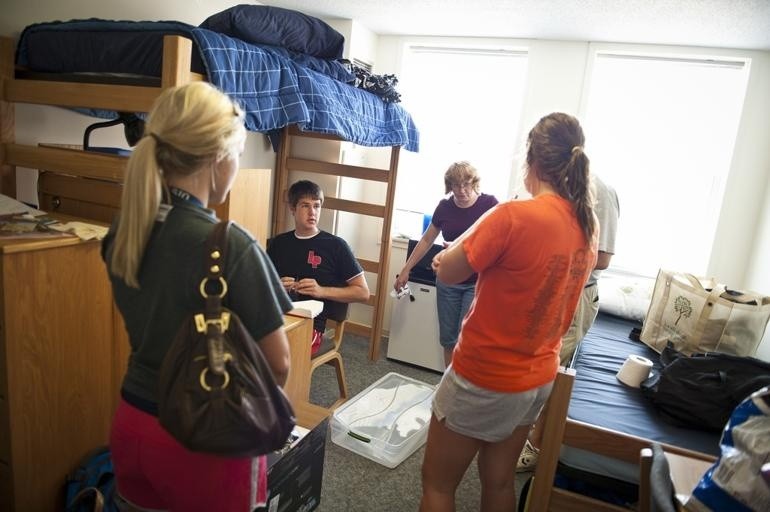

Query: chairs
<box><xmin>310</xmin><ymin>301</ymin><xmax>348</xmax><ymax>398</ymax></box>
<box><xmin>640</xmin><ymin>440</ymin><xmax>675</xmax><ymax>511</ymax></box>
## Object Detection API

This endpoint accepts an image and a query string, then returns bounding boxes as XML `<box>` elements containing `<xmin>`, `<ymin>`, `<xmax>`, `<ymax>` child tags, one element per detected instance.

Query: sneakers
<box><xmin>514</xmin><ymin>437</ymin><xmax>541</xmax><ymax>475</ymax></box>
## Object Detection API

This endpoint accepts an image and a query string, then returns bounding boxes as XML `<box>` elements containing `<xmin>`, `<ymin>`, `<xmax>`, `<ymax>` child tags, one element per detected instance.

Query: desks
<box><xmin>665</xmin><ymin>452</ymin><xmax>717</xmax><ymax>509</ymax></box>
<box><xmin>279</xmin><ymin>314</ymin><xmax>313</xmax><ymax>403</ymax></box>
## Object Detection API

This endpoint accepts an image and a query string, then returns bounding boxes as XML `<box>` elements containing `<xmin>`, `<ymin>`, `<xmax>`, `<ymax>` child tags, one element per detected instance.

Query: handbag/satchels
<box><xmin>639</xmin><ymin>350</ymin><xmax>770</xmax><ymax>434</ymax></box>
<box><xmin>157</xmin><ymin>219</ymin><xmax>298</xmax><ymax>461</ymax></box>
<box><xmin>638</xmin><ymin>263</ymin><xmax>770</xmax><ymax>365</ymax></box>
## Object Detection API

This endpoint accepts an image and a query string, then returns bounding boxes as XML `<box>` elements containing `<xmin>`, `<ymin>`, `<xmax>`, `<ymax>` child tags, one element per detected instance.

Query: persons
<box><xmin>266</xmin><ymin>179</ymin><xmax>371</xmax><ymax>353</ymax></box>
<box><xmin>404</xmin><ymin>112</ymin><xmax>601</xmax><ymax>512</ymax></box>
<box><xmin>510</xmin><ymin>164</ymin><xmax>622</xmax><ymax>474</ymax></box>
<box><xmin>392</xmin><ymin>160</ymin><xmax>501</xmax><ymax>368</ymax></box>
<box><xmin>93</xmin><ymin>79</ymin><xmax>292</xmax><ymax>511</ymax></box>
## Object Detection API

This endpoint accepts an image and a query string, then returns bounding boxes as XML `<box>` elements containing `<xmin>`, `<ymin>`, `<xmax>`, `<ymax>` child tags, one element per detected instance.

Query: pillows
<box><xmin>596</xmin><ymin>276</ymin><xmax>655</xmax><ymax>322</ymax></box>
<box><xmin>26</xmin><ymin>31</ymin><xmax>204</xmax><ymax>77</ymax></box>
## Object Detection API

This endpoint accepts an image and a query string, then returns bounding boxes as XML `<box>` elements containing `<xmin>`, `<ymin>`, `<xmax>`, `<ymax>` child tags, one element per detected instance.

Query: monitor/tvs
<box><xmin>406</xmin><ymin>239</ymin><xmax>445</xmax><ymax>286</ymax></box>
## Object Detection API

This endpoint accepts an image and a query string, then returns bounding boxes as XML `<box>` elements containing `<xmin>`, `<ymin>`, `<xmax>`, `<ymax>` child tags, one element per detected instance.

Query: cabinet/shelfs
<box><xmin>0</xmin><ymin>211</ymin><xmax>121</xmax><ymax>512</ymax></box>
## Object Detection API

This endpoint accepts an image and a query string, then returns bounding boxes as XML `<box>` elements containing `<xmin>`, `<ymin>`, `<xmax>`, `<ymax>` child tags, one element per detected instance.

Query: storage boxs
<box><xmin>265</xmin><ymin>400</ymin><xmax>333</xmax><ymax>512</ymax></box>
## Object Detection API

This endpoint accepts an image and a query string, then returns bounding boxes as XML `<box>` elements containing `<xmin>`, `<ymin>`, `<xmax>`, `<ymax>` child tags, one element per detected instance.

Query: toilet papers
<box><xmin>616</xmin><ymin>354</ymin><xmax>654</xmax><ymax>389</ymax></box>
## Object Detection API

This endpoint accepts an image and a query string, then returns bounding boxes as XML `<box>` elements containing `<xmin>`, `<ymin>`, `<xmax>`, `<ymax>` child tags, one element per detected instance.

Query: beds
<box><xmin>2</xmin><ymin>34</ymin><xmax>420</xmax><ymax>363</ymax></box>
<box><xmin>521</xmin><ymin>307</ymin><xmax>723</xmax><ymax>510</ymax></box>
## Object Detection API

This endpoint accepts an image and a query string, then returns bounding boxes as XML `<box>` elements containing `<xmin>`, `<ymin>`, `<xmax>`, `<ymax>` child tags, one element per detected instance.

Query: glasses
<box><xmin>450</xmin><ymin>182</ymin><xmax>475</xmax><ymax>189</ymax></box>
